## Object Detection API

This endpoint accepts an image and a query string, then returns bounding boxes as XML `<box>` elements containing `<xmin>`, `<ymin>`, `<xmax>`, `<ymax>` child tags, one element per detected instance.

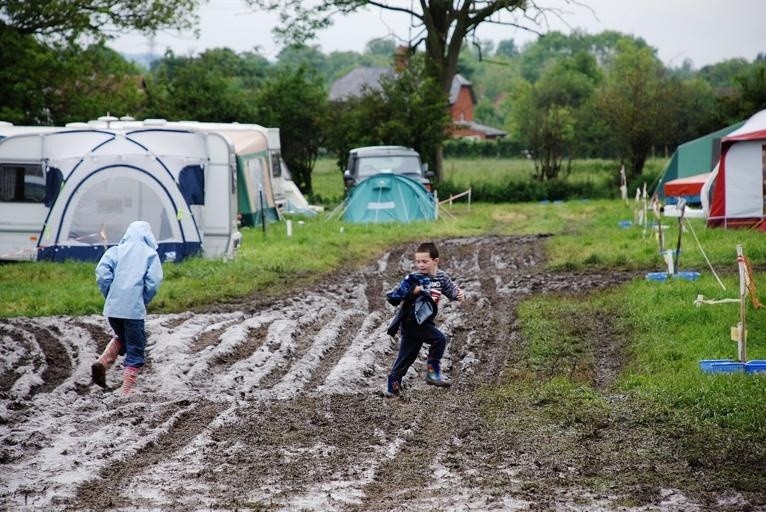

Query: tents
<box><xmin>707</xmin><ymin>108</ymin><xmax>766</xmax><ymax>231</ymax></box>
<box><xmin>337</xmin><ymin>170</ymin><xmax>440</xmax><ymax>222</ymax></box>
<box><xmin>1</xmin><ymin>116</ymin><xmax>322</xmax><ymax>262</ymax></box>
<box><xmin>648</xmin><ymin>118</ymin><xmax>748</xmax><ymax>211</ymax></box>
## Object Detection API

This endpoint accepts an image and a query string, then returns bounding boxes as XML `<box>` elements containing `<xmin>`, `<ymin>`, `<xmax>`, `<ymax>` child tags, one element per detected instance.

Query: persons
<box><xmin>383</xmin><ymin>242</ymin><xmax>465</xmax><ymax>395</ymax></box>
<box><xmin>89</xmin><ymin>220</ymin><xmax>162</xmax><ymax>396</ymax></box>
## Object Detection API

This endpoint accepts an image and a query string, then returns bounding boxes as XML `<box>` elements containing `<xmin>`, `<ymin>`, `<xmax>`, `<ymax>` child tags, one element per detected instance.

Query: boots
<box><xmin>122</xmin><ymin>366</ymin><xmax>140</xmax><ymax>395</ymax></box>
<box><xmin>425</xmin><ymin>353</ymin><xmax>451</xmax><ymax>387</ymax></box>
<box><xmin>91</xmin><ymin>334</ymin><xmax>122</xmax><ymax>388</ymax></box>
<box><xmin>387</xmin><ymin>374</ymin><xmax>401</xmax><ymax>395</ymax></box>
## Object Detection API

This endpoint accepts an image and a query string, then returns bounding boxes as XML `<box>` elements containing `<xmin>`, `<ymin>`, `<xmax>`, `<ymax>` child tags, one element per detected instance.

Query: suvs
<box><xmin>344</xmin><ymin>143</ymin><xmax>435</xmax><ymax>206</ymax></box>
